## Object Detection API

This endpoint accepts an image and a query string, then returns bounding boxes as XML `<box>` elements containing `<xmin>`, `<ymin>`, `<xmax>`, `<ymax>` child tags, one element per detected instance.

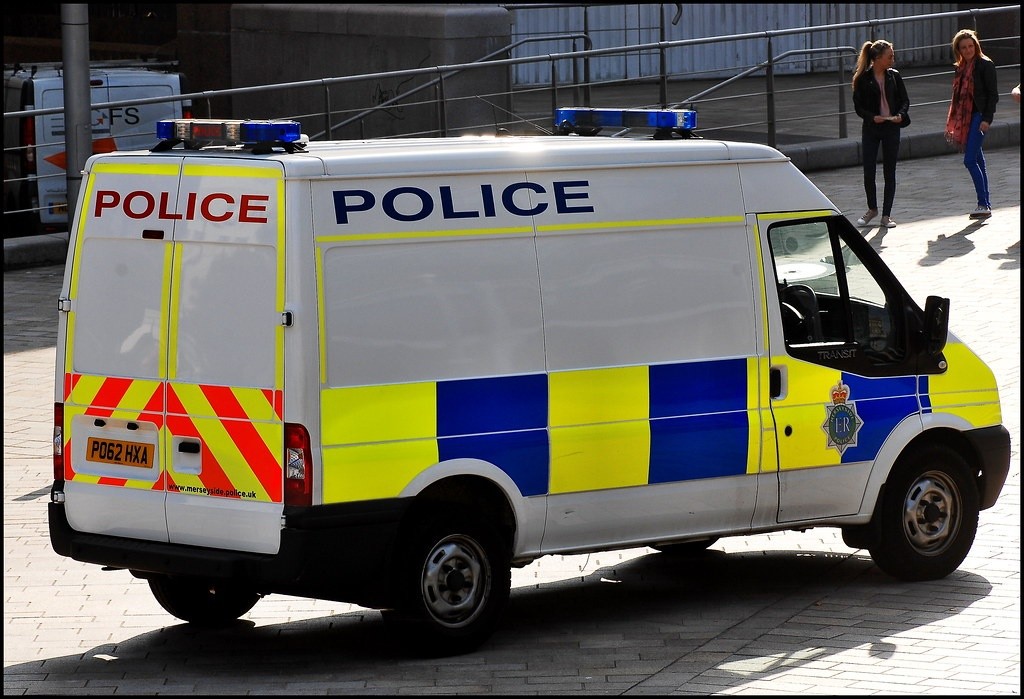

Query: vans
<box><xmin>47</xmin><ymin>97</ymin><xmax>1015</xmax><ymax>653</ymax></box>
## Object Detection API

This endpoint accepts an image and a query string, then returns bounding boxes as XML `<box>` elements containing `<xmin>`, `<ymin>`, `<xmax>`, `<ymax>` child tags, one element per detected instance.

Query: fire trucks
<box><xmin>0</xmin><ymin>60</ymin><xmax>197</xmax><ymax>230</ymax></box>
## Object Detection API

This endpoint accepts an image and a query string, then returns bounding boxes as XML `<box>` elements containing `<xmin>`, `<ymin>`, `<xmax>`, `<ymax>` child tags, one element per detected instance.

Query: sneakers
<box><xmin>880</xmin><ymin>216</ymin><xmax>896</xmax><ymax>227</ymax></box>
<box><xmin>969</xmin><ymin>206</ymin><xmax>992</xmax><ymax>219</ymax></box>
<box><xmin>858</xmin><ymin>208</ymin><xmax>879</xmax><ymax>225</ymax></box>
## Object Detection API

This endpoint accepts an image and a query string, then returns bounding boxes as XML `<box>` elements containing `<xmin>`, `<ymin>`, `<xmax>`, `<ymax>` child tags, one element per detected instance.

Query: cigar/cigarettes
<box><xmin>981</xmin><ymin>130</ymin><xmax>984</xmax><ymax>135</ymax></box>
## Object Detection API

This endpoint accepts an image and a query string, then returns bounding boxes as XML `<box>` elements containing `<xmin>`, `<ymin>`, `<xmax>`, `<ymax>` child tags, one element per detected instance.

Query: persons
<box><xmin>1012</xmin><ymin>84</ymin><xmax>1020</xmax><ymax>102</ymax></box>
<box><xmin>852</xmin><ymin>40</ymin><xmax>910</xmax><ymax>227</ymax></box>
<box><xmin>944</xmin><ymin>29</ymin><xmax>999</xmax><ymax>219</ymax></box>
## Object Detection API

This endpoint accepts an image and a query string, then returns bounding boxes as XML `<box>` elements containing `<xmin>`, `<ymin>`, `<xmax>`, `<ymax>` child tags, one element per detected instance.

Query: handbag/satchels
<box><xmin>889</xmin><ymin>67</ymin><xmax>911</xmax><ymax>128</ymax></box>
